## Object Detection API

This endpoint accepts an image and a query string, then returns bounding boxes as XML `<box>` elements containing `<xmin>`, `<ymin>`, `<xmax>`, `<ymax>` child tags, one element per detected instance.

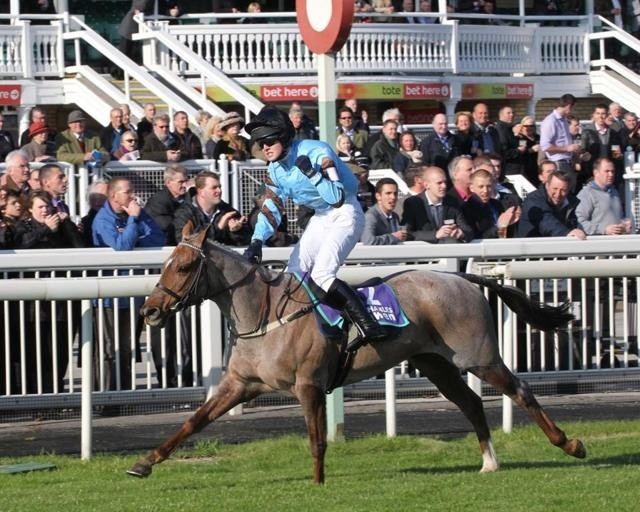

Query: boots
<box><xmin>327</xmin><ymin>278</ymin><xmax>379</xmax><ymax>351</ymax></box>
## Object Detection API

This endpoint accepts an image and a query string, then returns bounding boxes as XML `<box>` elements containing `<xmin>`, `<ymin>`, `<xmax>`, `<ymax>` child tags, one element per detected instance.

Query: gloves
<box><xmin>295</xmin><ymin>156</ymin><xmax>318</xmax><ymax>178</ymax></box>
<box><xmin>243</xmin><ymin>239</ymin><xmax>262</xmax><ymax>264</ymax></box>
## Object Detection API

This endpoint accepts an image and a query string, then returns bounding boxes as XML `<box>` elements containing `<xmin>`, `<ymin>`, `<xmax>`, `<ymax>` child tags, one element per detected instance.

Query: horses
<box><xmin>125</xmin><ymin>219</ymin><xmax>586</xmax><ymax>485</ymax></box>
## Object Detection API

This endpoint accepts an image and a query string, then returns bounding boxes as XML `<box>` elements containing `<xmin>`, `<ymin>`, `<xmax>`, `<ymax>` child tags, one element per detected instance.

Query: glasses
<box><xmin>127</xmin><ymin>139</ymin><xmax>139</xmax><ymax>143</ymax></box>
<box><xmin>256</xmin><ymin>133</ymin><xmax>283</xmax><ymax>150</ymax></box>
<box><xmin>160</xmin><ymin>126</ymin><xmax>169</xmax><ymax>128</ymax></box>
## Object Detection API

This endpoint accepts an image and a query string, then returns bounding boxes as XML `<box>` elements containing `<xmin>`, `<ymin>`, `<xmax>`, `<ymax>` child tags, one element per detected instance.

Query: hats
<box><xmin>220</xmin><ymin>112</ymin><xmax>245</xmax><ymax>131</ymax></box>
<box><xmin>28</xmin><ymin>122</ymin><xmax>50</xmax><ymax>137</ymax></box>
<box><xmin>68</xmin><ymin>111</ymin><xmax>87</xmax><ymax>123</ymax></box>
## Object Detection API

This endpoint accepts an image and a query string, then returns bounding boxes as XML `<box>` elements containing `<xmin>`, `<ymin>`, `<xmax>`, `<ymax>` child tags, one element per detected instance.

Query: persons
<box><xmin>1</xmin><ymin>93</ymin><xmax>640</xmax><ymax>372</ymax></box>
<box><xmin>108</xmin><ymin>0</ymin><xmax>640</xmax><ymax>81</ymax></box>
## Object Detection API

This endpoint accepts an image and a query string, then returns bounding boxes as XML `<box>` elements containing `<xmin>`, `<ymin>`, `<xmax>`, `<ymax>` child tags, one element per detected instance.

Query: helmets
<box><xmin>245</xmin><ymin>105</ymin><xmax>295</xmax><ymax>140</ymax></box>
